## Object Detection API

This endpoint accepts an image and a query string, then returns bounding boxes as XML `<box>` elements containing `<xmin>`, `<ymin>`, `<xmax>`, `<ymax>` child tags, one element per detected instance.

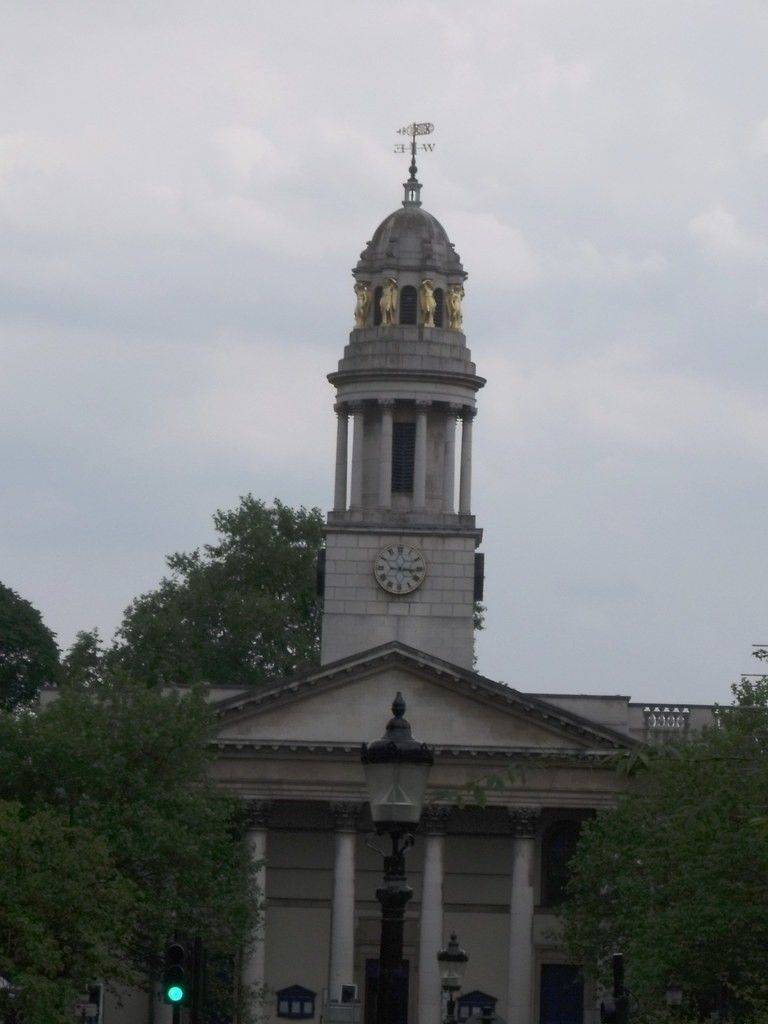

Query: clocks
<box><xmin>374</xmin><ymin>543</ymin><xmax>427</xmax><ymax>595</ymax></box>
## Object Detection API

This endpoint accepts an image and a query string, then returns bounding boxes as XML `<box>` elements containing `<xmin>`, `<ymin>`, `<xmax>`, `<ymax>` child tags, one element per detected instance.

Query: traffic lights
<box><xmin>159</xmin><ymin>927</ymin><xmax>195</xmax><ymax>1008</ymax></box>
<box><xmin>190</xmin><ymin>933</ymin><xmax>209</xmax><ymax>1014</ymax></box>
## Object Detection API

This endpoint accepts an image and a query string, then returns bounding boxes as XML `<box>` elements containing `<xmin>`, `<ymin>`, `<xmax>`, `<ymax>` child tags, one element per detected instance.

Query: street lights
<box><xmin>359</xmin><ymin>692</ymin><xmax>438</xmax><ymax>1023</ymax></box>
<box><xmin>435</xmin><ymin>931</ymin><xmax>470</xmax><ymax>1023</ymax></box>
